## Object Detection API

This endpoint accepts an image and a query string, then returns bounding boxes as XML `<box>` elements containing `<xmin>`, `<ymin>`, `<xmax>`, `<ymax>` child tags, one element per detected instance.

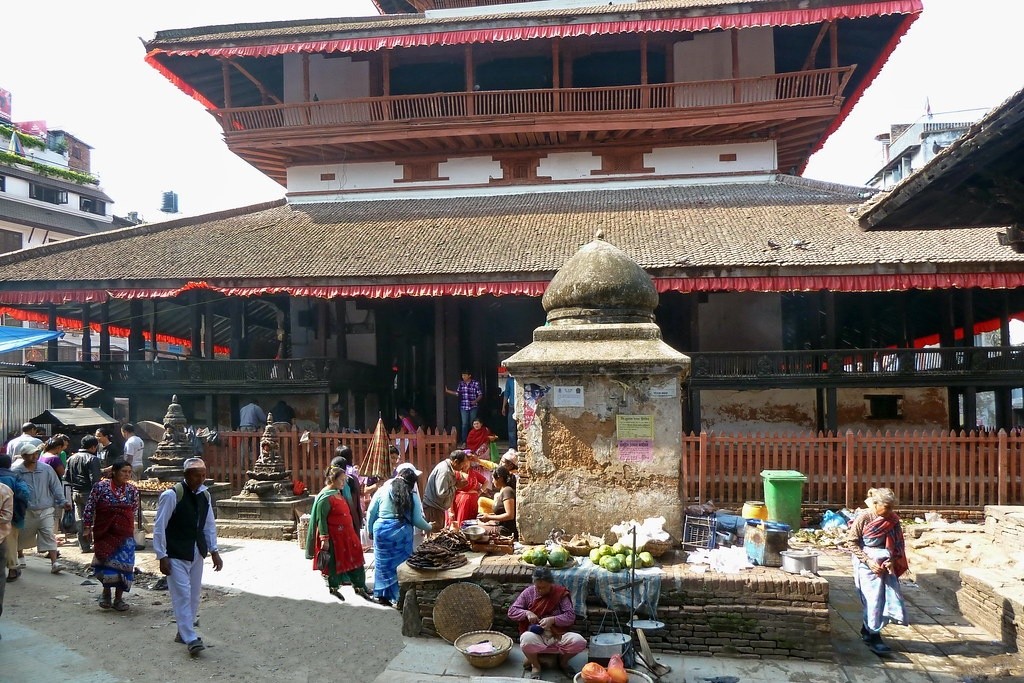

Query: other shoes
<box><xmin>860</xmin><ymin>624</ymin><xmax>892</xmax><ymax>654</ymax></box>
<box><xmin>460</xmin><ymin>443</ymin><xmax>466</xmax><ymax>450</ymax></box>
<box><xmin>374</xmin><ymin>596</ymin><xmax>403</xmax><ymax>610</ymax></box>
<box><xmin>15</xmin><ymin>530</ymin><xmax>95</xmax><ymax>557</ymax></box>
<box><xmin>7</xmin><ymin>569</ymin><xmax>22</xmax><ymax>582</ymax></box>
<box><xmin>322</xmin><ymin>573</ymin><xmax>376</xmax><ymax>602</ymax></box>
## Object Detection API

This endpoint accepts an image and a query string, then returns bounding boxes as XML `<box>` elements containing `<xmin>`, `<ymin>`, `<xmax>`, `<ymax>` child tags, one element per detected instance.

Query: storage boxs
<box><xmin>743</xmin><ymin>522</ymin><xmax>789</xmax><ymax>568</ymax></box>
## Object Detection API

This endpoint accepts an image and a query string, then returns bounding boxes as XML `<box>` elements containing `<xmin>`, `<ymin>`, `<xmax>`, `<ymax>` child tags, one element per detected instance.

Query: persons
<box><xmin>331</xmin><ymin>444</ymin><xmax>364</xmax><ymax>489</ymax></box>
<box><xmin>466</xmin><ymin>448</ymin><xmax>517</xmax><ymax>498</ymax></box>
<box><xmin>120</xmin><ymin>422</ymin><xmax>150</xmax><ymax>532</ymax></box>
<box><xmin>363</xmin><ymin>444</ymin><xmax>400</xmax><ymax>505</ymax></box>
<box><xmin>389</xmin><ymin>369</ymin><xmax>517</xmax><ymax>494</ymax></box>
<box><xmin>506</xmin><ymin>567</ymin><xmax>587</xmax><ymax>680</ymax></box>
<box><xmin>94</xmin><ymin>428</ymin><xmax>119</xmax><ymax>479</ymax></box>
<box><xmin>476</xmin><ymin>465</ymin><xmax>517</xmax><ymax>541</ymax></box>
<box><xmin>323</xmin><ymin>456</ymin><xmax>364</xmax><ymax>540</ymax></box>
<box><xmin>351</xmin><ymin>463</ymin><xmax>373</xmax><ymax>554</ymax></box>
<box><xmin>395</xmin><ymin>461</ymin><xmax>428</xmax><ymax>553</ymax></box>
<box><xmin>0</xmin><ymin>454</ymin><xmax>28</xmax><ymax>582</ymax></box>
<box><xmin>65</xmin><ymin>434</ymin><xmax>103</xmax><ymax>554</ymax></box>
<box><xmin>83</xmin><ymin>459</ymin><xmax>140</xmax><ymax>612</ymax></box>
<box><xmin>13</xmin><ymin>444</ymin><xmax>72</xmax><ymax>574</ymax></box>
<box><xmin>449</xmin><ymin>451</ymin><xmax>489</xmax><ymax>530</ymax></box>
<box><xmin>368</xmin><ymin>468</ymin><xmax>436</xmax><ymax>606</ymax></box>
<box><xmin>0</xmin><ymin>423</ymin><xmax>74</xmax><ymax>547</ymax></box>
<box><xmin>846</xmin><ymin>486</ymin><xmax>909</xmax><ymax>657</ymax></box>
<box><xmin>0</xmin><ymin>482</ymin><xmax>15</xmax><ymax>620</ymax></box>
<box><xmin>237</xmin><ymin>398</ymin><xmax>268</xmax><ymax>470</ymax></box>
<box><xmin>422</xmin><ymin>449</ymin><xmax>469</xmax><ymax>533</ymax></box>
<box><xmin>303</xmin><ymin>466</ymin><xmax>371</xmax><ymax>601</ymax></box>
<box><xmin>266</xmin><ymin>399</ymin><xmax>297</xmax><ymax>459</ymax></box>
<box><xmin>153</xmin><ymin>458</ymin><xmax>223</xmax><ymax>656</ymax></box>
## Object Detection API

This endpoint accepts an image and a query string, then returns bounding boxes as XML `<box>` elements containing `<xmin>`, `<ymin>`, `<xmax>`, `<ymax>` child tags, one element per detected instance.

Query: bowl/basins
<box><xmin>463</xmin><ymin>526</ymin><xmax>485</xmax><ymax>541</ymax></box>
<box><xmin>779</xmin><ymin>550</ymin><xmax>820</xmax><ymax>573</ymax></box>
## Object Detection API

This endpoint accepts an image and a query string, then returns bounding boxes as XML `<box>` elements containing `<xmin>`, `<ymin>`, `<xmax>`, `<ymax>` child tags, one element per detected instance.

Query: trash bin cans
<box><xmin>758</xmin><ymin>468</ymin><xmax>807</xmax><ymax>534</ymax></box>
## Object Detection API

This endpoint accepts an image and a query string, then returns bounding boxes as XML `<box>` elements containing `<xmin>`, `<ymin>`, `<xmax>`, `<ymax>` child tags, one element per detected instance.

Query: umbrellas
<box><xmin>359</xmin><ymin>410</ymin><xmax>393</xmax><ymax>487</ymax></box>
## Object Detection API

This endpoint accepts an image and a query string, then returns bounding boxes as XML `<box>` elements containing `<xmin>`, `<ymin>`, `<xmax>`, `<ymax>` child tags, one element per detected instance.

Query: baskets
<box><xmin>297</xmin><ymin>523</ymin><xmax>309</xmax><ymax>550</ymax></box>
<box><xmin>452</xmin><ymin>630</ymin><xmax>514</xmax><ymax>668</ymax></box>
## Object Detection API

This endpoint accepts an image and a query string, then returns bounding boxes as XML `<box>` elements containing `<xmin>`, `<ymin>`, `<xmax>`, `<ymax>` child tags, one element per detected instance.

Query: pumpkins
<box><xmin>590</xmin><ymin>542</ymin><xmax>654</xmax><ymax>571</ymax></box>
<box><xmin>522</xmin><ymin>543</ymin><xmax>570</xmax><ymax>566</ymax></box>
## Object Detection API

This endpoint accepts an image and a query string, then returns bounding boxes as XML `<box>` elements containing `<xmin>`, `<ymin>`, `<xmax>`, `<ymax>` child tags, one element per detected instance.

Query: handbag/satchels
<box><xmin>490</xmin><ymin>439</ymin><xmax>499</xmax><ymax>462</ymax></box>
<box><xmin>61</xmin><ymin>508</ymin><xmax>79</xmax><ymax>533</ymax></box>
<box><xmin>529</xmin><ymin>623</ymin><xmax>544</xmax><ymax>634</ymax></box>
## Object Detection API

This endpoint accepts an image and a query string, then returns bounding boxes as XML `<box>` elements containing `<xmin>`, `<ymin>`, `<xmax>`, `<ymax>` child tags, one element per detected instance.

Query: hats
<box><xmin>21</xmin><ymin>443</ymin><xmax>40</xmax><ymax>456</ymax></box>
<box><xmin>183</xmin><ymin>458</ymin><xmax>207</xmax><ymax>472</ymax></box>
<box><xmin>503</xmin><ymin>448</ymin><xmax>519</xmax><ymax>466</ymax></box>
<box><xmin>396</xmin><ymin>463</ymin><xmax>423</xmax><ymax>476</ymax></box>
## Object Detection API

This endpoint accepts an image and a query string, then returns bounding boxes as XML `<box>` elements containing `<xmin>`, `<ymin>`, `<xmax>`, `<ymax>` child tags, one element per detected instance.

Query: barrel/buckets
<box><xmin>742</xmin><ymin>500</ymin><xmax>768</xmax><ymax>522</ymax></box>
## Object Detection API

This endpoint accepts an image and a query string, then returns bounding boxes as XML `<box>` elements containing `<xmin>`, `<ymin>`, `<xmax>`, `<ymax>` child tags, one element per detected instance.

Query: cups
<box><xmin>449</xmin><ymin>520</ymin><xmax>459</xmax><ymax>532</ymax></box>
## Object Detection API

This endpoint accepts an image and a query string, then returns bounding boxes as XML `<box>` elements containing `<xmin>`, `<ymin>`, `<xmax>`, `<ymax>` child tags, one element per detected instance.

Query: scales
<box><xmin>592</xmin><ymin>577</ymin><xmax>664</xmax><ymax>646</ymax></box>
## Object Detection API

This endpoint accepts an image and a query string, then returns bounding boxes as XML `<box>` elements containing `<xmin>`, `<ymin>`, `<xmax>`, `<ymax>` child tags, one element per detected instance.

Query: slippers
<box><xmin>561</xmin><ymin>665</ymin><xmax>576</xmax><ymax>678</ymax></box>
<box><xmin>531</xmin><ymin>664</ymin><xmax>542</xmax><ymax>680</ymax></box>
<box><xmin>175</xmin><ymin>632</ymin><xmax>204</xmax><ymax>656</ymax></box>
<box><xmin>16</xmin><ymin>557</ymin><xmax>25</xmax><ymax>570</ymax></box>
<box><xmin>52</xmin><ymin>562</ymin><xmax>66</xmax><ymax>573</ymax></box>
<box><xmin>97</xmin><ymin>593</ymin><xmax>129</xmax><ymax>611</ymax></box>
